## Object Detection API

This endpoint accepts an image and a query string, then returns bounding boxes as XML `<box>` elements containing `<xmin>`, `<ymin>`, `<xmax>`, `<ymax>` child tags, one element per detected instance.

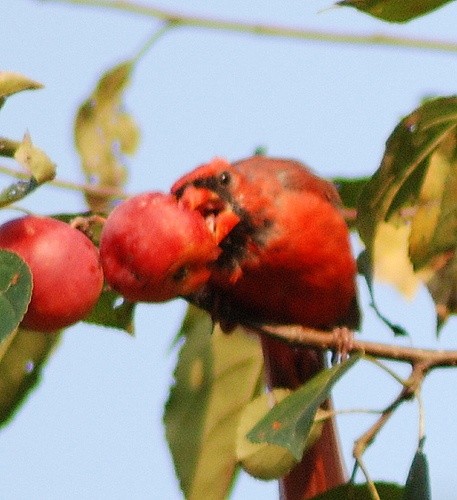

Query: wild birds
<box><xmin>170</xmin><ymin>155</ymin><xmax>360</xmax><ymax>500</ymax></box>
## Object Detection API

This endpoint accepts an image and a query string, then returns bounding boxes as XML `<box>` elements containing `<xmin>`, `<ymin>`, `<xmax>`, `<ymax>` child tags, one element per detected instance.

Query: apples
<box><xmin>99</xmin><ymin>192</ymin><xmax>217</xmax><ymax>300</ymax></box>
<box><xmin>1</xmin><ymin>216</ymin><xmax>104</xmax><ymax>332</ymax></box>
